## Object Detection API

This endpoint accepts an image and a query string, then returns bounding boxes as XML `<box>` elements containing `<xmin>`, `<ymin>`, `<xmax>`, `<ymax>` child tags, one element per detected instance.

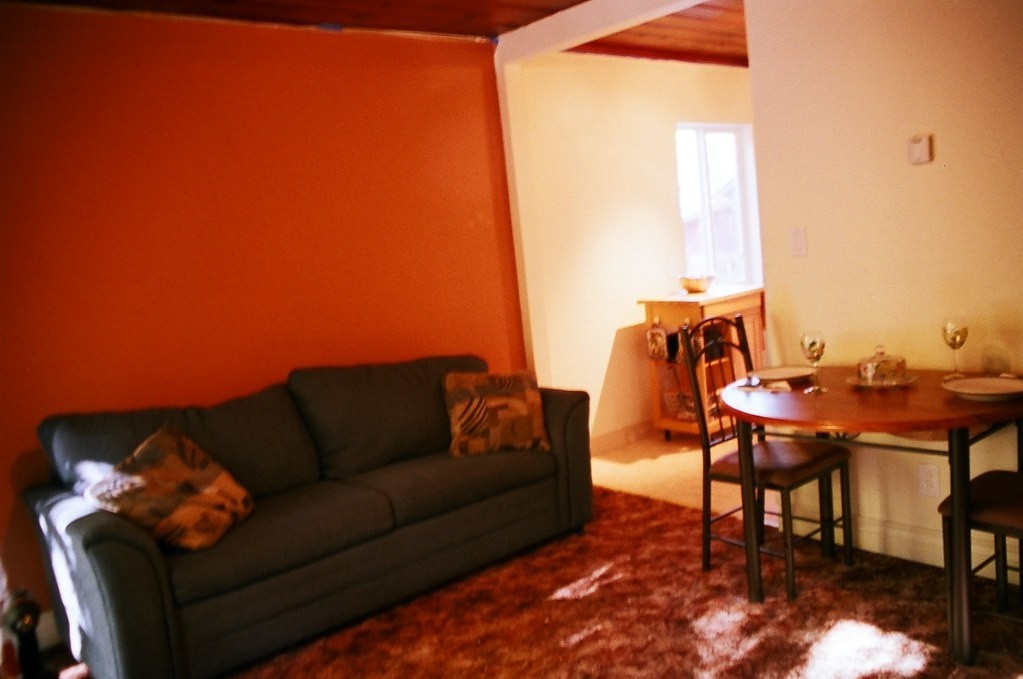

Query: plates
<box><xmin>943</xmin><ymin>377</ymin><xmax>1023</xmax><ymax>404</ymax></box>
<box><xmin>747</xmin><ymin>367</ymin><xmax>816</xmax><ymax>386</ymax></box>
<box><xmin>844</xmin><ymin>374</ymin><xmax>919</xmax><ymax>390</ymax></box>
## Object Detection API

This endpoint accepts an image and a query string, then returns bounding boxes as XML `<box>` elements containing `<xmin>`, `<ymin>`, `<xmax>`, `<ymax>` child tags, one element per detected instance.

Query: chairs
<box><xmin>679</xmin><ymin>314</ymin><xmax>852</xmax><ymax>603</ymax></box>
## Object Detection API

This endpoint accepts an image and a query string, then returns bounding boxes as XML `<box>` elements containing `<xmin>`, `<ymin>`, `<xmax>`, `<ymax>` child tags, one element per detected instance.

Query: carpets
<box><xmin>225</xmin><ymin>484</ymin><xmax>1023</xmax><ymax>678</ymax></box>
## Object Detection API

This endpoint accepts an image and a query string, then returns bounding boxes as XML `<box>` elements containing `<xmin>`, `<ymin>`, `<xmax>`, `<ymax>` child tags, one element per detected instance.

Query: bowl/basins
<box><xmin>679</xmin><ymin>273</ymin><xmax>714</xmax><ymax>292</ymax></box>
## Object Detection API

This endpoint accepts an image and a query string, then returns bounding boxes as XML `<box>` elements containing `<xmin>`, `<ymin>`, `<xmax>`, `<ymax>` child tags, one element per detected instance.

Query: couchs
<box><xmin>20</xmin><ymin>357</ymin><xmax>592</xmax><ymax>679</ymax></box>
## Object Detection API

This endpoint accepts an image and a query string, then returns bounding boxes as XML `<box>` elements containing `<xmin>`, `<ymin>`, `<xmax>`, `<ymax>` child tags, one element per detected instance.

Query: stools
<box><xmin>938</xmin><ymin>469</ymin><xmax>1023</xmax><ymax>658</ymax></box>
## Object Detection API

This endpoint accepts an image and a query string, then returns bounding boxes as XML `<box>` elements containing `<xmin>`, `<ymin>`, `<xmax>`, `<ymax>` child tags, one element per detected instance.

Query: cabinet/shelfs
<box><xmin>641</xmin><ymin>284</ymin><xmax>768</xmax><ymax>445</ymax></box>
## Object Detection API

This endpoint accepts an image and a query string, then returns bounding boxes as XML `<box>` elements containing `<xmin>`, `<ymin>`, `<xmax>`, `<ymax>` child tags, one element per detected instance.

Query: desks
<box><xmin>718</xmin><ymin>366</ymin><xmax>1022</xmax><ymax>663</ymax></box>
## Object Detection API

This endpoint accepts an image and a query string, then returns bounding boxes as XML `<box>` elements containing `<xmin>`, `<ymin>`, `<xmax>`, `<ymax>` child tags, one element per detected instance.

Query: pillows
<box><xmin>84</xmin><ymin>427</ymin><xmax>252</xmax><ymax>552</ymax></box>
<box><xmin>444</xmin><ymin>365</ymin><xmax>552</xmax><ymax>456</ymax></box>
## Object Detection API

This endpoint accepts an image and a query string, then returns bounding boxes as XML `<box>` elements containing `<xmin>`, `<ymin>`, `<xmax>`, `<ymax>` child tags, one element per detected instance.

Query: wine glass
<box><xmin>799</xmin><ymin>329</ymin><xmax>829</xmax><ymax>396</ymax></box>
<box><xmin>941</xmin><ymin>320</ymin><xmax>969</xmax><ymax>385</ymax></box>
